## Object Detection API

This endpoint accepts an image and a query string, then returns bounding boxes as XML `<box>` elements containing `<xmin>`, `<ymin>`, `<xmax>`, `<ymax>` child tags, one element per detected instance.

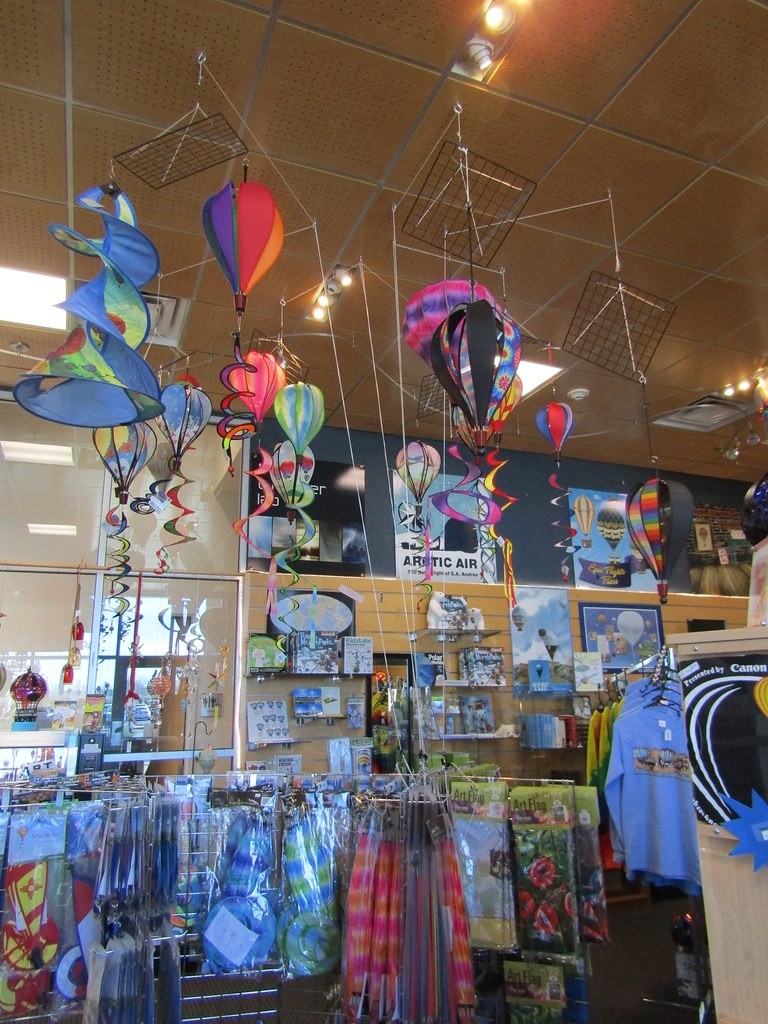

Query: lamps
<box><xmin>461</xmin><ymin>32</ymin><xmax>495</xmax><ymax>71</ymax></box>
<box><xmin>327</xmin><ymin>280</ymin><xmax>342</xmax><ymax>294</ymax></box>
<box><xmin>483</xmin><ymin>3</ymin><xmax>515</xmax><ymax>36</ymax></box>
<box><xmin>317</xmin><ymin>290</ymin><xmax>337</xmax><ymax>307</ymax></box>
<box><xmin>724</xmin><ymin>386</ymin><xmax>734</xmax><ymax>396</ymax></box>
<box><xmin>738</xmin><ymin>381</ymin><xmax>750</xmax><ymax>391</ymax></box>
<box><xmin>332</xmin><ymin>266</ymin><xmax>353</xmax><ymax>286</ymax></box>
<box><xmin>313</xmin><ymin>305</ymin><xmax>328</xmax><ymax>322</ymax></box>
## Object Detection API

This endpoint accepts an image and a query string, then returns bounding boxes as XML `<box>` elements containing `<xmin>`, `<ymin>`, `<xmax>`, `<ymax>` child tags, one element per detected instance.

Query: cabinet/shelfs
<box><xmin>696</xmin><ymin>820</ymin><xmax>768</xmax><ymax>1024</ymax></box>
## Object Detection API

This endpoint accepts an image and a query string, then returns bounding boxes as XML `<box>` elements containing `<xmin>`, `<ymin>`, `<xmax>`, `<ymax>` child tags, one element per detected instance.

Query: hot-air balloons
<box><xmin>629</xmin><ymin>538</ymin><xmax>647</xmax><ymax>574</ymax></box>
<box><xmin>512</xmin><ymin>605</ymin><xmax>528</xmax><ymax>630</ymax></box>
<box><xmin>573</xmin><ymin>495</ymin><xmax>594</xmax><ymax>548</ymax></box>
<box><xmin>597</xmin><ymin>503</ymin><xmax>625</xmax><ymax>566</ymax></box>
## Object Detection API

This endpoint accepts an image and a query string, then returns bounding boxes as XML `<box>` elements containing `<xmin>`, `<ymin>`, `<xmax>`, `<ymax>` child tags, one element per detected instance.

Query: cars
<box><xmin>105</xmin><ymin>700</ymin><xmax>160</xmax><ymax>726</ymax></box>
